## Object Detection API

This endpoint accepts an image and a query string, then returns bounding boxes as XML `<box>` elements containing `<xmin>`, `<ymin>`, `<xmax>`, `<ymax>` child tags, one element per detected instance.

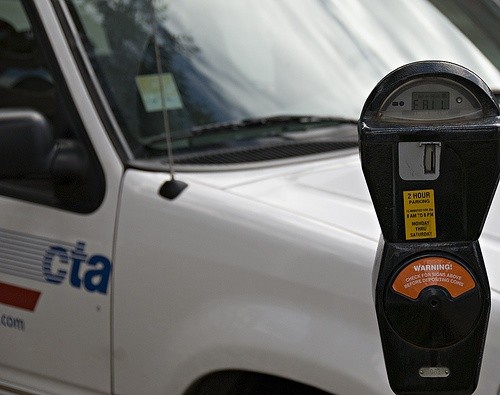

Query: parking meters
<box><xmin>357</xmin><ymin>60</ymin><xmax>500</xmax><ymax>395</ymax></box>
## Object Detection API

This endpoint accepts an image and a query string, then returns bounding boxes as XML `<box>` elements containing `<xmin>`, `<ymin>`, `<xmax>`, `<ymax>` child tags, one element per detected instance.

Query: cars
<box><xmin>0</xmin><ymin>0</ymin><xmax>499</xmax><ymax>394</ymax></box>
